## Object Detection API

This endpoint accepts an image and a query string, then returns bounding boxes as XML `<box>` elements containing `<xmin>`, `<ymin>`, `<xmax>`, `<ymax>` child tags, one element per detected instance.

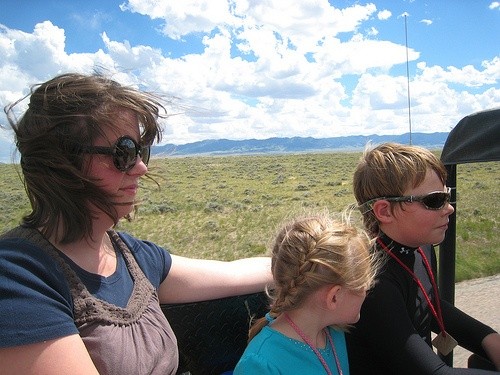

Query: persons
<box><xmin>0</xmin><ymin>71</ymin><xmax>275</xmax><ymax>375</ymax></box>
<box><xmin>344</xmin><ymin>142</ymin><xmax>500</xmax><ymax>375</ymax></box>
<box><xmin>234</xmin><ymin>211</ymin><xmax>389</xmax><ymax>375</ymax></box>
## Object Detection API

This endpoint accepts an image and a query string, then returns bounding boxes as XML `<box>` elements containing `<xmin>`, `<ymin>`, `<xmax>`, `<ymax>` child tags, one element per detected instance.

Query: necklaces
<box><xmin>372</xmin><ymin>233</ymin><xmax>458</xmax><ymax>356</ymax></box>
<box><xmin>282</xmin><ymin>312</ymin><xmax>342</xmax><ymax>375</ymax></box>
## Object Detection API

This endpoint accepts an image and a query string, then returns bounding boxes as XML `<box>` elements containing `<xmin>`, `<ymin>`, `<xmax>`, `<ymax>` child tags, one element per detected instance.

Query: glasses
<box><xmin>360</xmin><ymin>186</ymin><xmax>452</xmax><ymax>216</ymax></box>
<box><xmin>58</xmin><ymin>134</ymin><xmax>151</xmax><ymax>172</ymax></box>
